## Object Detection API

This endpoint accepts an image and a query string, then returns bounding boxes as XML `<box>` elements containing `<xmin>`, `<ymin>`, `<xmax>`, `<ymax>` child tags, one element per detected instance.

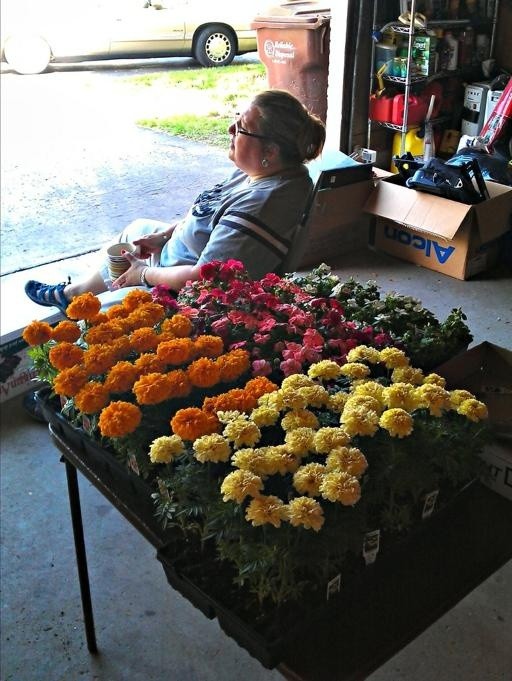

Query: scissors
<box><xmin>397</xmin><ymin>12</ymin><xmax>436</xmax><ymax>37</ymax></box>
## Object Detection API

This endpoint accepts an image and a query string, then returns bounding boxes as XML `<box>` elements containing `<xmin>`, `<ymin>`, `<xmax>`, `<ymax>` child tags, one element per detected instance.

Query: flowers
<box><xmin>23</xmin><ymin>258</ymin><xmax>491</xmax><ymax>603</ymax></box>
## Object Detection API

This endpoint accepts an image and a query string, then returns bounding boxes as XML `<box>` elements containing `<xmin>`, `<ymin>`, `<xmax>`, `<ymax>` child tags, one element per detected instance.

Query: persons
<box><xmin>20</xmin><ymin>85</ymin><xmax>327</xmax><ymax>423</ymax></box>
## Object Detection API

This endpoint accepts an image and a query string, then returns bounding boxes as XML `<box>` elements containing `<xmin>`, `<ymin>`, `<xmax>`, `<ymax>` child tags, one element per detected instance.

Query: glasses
<box><xmin>233</xmin><ymin>110</ymin><xmax>274</xmax><ymax>141</ymax></box>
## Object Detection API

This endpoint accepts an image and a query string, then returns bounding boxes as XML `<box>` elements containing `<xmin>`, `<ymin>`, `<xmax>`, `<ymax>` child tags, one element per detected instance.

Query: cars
<box><xmin>1</xmin><ymin>0</ymin><xmax>258</xmax><ymax>78</ymax></box>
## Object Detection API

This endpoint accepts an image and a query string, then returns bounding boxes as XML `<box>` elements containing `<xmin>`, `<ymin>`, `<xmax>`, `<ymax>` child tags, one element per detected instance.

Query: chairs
<box><xmin>283</xmin><ymin>165</ymin><xmax>321</xmax><ymax>279</ymax></box>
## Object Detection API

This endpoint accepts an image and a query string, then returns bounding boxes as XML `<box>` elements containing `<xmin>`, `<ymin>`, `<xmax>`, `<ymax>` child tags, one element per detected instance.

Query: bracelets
<box><xmin>140</xmin><ymin>266</ymin><xmax>155</xmax><ymax>288</ymax></box>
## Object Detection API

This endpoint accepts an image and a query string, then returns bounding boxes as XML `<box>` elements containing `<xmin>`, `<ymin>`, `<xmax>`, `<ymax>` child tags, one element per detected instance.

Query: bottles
<box><xmin>393</xmin><ymin>57</ymin><xmax>409</xmax><ymax>78</ymax></box>
<box><xmin>428</xmin><ymin>26</ymin><xmax>475</xmax><ymax>75</ymax></box>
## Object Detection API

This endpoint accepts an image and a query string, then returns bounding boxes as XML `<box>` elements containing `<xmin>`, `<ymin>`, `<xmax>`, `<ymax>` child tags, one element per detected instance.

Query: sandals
<box><xmin>25</xmin><ymin>277</ymin><xmax>81</xmax><ymax>321</ymax></box>
<box><xmin>22</xmin><ymin>392</ymin><xmax>47</xmax><ymax>423</ymax></box>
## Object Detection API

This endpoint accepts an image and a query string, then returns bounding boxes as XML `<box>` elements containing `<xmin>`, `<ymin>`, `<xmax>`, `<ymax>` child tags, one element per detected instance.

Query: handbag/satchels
<box><xmin>406</xmin><ymin>158</ymin><xmax>481</xmax><ymax>204</ymax></box>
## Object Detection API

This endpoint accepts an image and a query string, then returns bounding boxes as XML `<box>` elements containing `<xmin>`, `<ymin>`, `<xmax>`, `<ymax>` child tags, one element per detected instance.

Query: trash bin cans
<box><xmin>249</xmin><ymin>14</ymin><xmax>332</xmax><ymax>124</ymax></box>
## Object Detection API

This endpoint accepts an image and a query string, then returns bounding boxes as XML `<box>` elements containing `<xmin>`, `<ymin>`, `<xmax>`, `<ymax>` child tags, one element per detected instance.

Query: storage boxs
<box><xmin>413</xmin><ymin>341</ymin><xmax>512</xmax><ymax>501</ymax></box>
<box><xmin>289</xmin><ymin>151</ymin><xmax>512</xmax><ymax>281</ymax></box>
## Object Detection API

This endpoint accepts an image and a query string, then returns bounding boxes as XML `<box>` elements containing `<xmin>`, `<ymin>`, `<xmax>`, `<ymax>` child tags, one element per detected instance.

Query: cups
<box><xmin>106</xmin><ymin>241</ymin><xmax>137</xmax><ymax>283</ymax></box>
